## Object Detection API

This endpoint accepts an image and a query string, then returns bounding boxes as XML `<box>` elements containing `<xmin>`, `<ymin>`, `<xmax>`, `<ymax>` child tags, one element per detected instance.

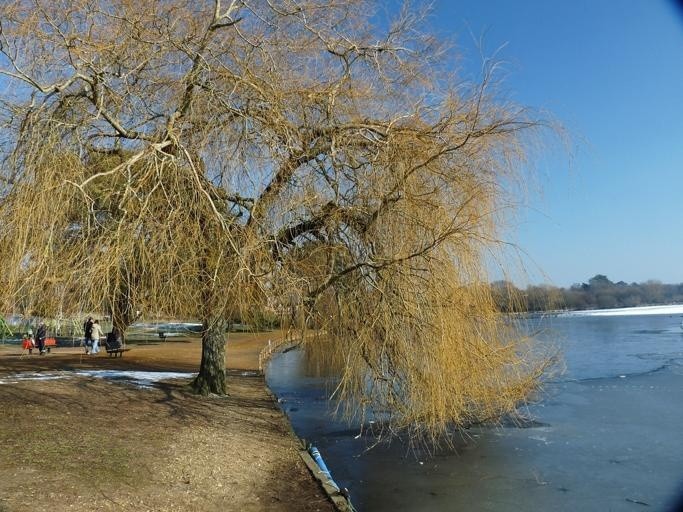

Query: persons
<box><xmin>89</xmin><ymin>319</ymin><xmax>104</xmax><ymax>354</ymax></box>
<box><xmin>83</xmin><ymin>316</ymin><xmax>94</xmax><ymax>355</ymax></box>
<box><xmin>35</xmin><ymin>321</ymin><xmax>48</xmax><ymax>356</ymax></box>
<box><xmin>26</xmin><ymin>333</ymin><xmax>36</xmax><ymax>354</ymax></box>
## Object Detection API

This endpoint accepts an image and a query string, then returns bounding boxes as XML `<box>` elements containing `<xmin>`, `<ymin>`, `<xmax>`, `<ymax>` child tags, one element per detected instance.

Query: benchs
<box><xmin>29</xmin><ymin>338</ymin><xmax>57</xmax><ymax>354</ymax></box>
<box><xmin>106</xmin><ymin>333</ymin><xmax>130</xmax><ymax>358</ymax></box>
